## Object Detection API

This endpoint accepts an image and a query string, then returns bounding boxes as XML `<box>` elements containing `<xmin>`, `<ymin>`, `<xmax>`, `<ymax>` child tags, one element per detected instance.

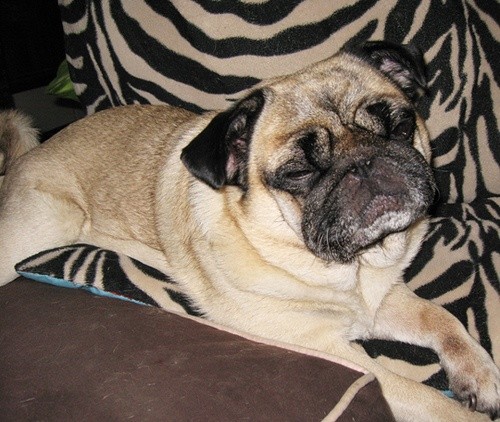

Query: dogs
<box><xmin>0</xmin><ymin>40</ymin><xmax>499</xmax><ymax>422</ymax></box>
